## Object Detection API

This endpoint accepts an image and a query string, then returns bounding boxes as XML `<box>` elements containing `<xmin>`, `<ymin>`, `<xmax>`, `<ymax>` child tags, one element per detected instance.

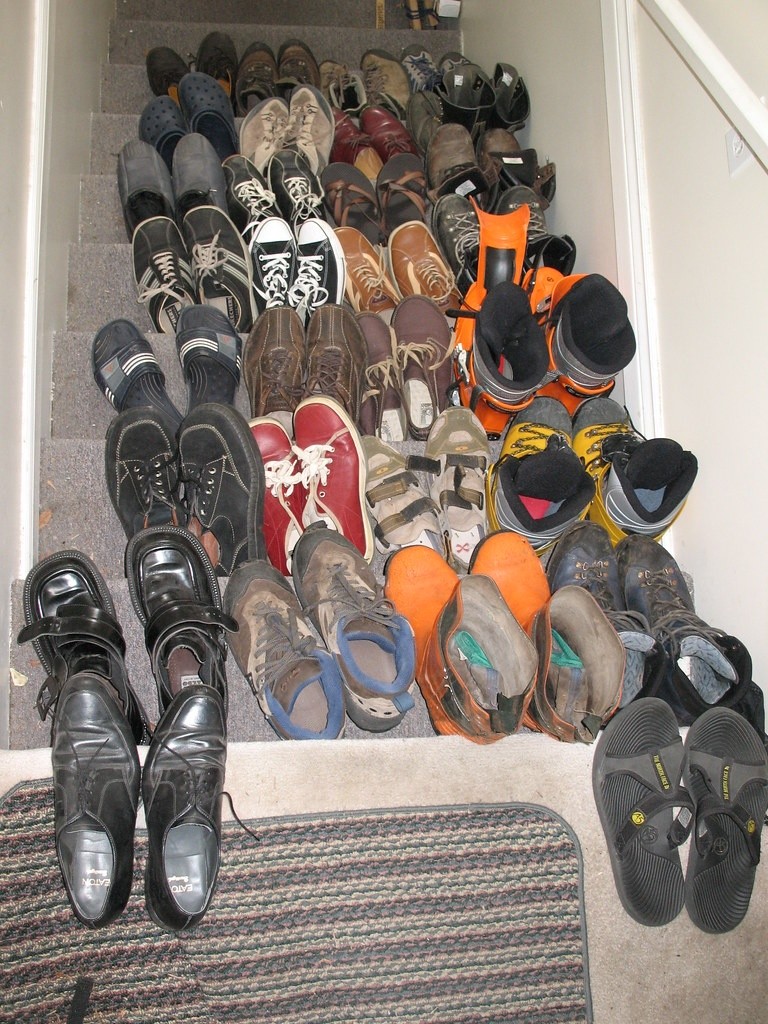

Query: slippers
<box><xmin>682</xmin><ymin>705</ymin><xmax>768</xmax><ymax>934</ymax></box>
<box><xmin>591</xmin><ymin>697</ymin><xmax>696</xmax><ymax>928</ymax></box>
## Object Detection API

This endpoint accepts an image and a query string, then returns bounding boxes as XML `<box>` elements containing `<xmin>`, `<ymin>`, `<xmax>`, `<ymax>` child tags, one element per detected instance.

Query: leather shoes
<box><xmin>140</xmin><ymin>683</ymin><xmax>260</xmax><ymax>931</ymax></box>
<box><xmin>50</xmin><ymin>673</ymin><xmax>142</xmax><ymax>926</ymax></box>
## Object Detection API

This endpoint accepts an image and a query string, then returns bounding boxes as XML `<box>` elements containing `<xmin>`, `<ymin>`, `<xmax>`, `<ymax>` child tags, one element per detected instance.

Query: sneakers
<box><xmin>11</xmin><ymin>25</ymin><xmax>758</xmax><ymax>746</ymax></box>
